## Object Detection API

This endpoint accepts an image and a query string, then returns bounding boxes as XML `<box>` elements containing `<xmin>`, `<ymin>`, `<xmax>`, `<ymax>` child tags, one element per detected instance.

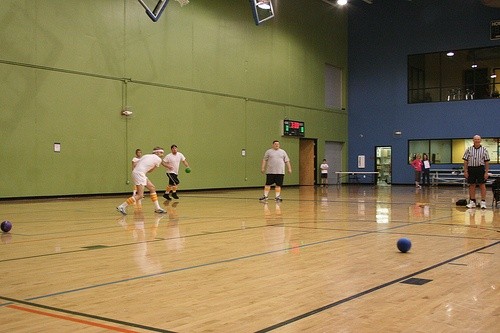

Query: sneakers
<box><xmin>274</xmin><ymin>196</ymin><xmax>282</xmax><ymax>202</ymax></box>
<box><xmin>171</xmin><ymin>192</ymin><xmax>179</xmax><ymax>199</ymax></box>
<box><xmin>259</xmin><ymin>196</ymin><xmax>268</xmax><ymax>201</ymax></box>
<box><xmin>163</xmin><ymin>194</ymin><xmax>172</xmax><ymax>201</ymax></box>
<box><xmin>155</xmin><ymin>208</ymin><xmax>167</xmax><ymax>214</ymax></box>
<box><xmin>480</xmin><ymin>201</ymin><xmax>486</xmax><ymax>209</ymax></box>
<box><xmin>115</xmin><ymin>206</ymin><xmax>127</xmax><ymax>216</ymax></box>
<box><xmin>465</xmin><ymin>200</ymin><xmax>476</xmax><ymax>208</ymax></box>
<box><xmin>416</xmin><ymin>184</ymin><xmax>422</xmax><ymax>188</ymax></box>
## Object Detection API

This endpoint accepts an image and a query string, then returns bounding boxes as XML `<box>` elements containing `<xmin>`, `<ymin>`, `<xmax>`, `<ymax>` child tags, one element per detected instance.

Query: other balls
<box><xmin>397</xmin><ymin>238</ymin><xmax>412</xmax><ymax>252</ymax></box>
<box><xmin>1</xmin><ymin>221</ymin><xmax>12</xmax><ymax>232</ymax></box>
<box><xmin>185</xmin><ymin>167</ymin><xmax>191</xmax><ymax>173</ymax></box>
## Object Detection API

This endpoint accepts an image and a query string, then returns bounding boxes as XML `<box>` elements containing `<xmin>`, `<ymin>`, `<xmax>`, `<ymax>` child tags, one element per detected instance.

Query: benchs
<box><xmin>420</xmin><ymin>168</ymin><xmax>500</xmax><ymax>189</ymax></box>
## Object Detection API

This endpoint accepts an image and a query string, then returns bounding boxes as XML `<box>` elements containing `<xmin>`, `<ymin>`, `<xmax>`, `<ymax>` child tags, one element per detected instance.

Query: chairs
<box><xmin>492</xmin><ymin>189</ymin><xmax>500</xmax><ymax>207</ymax></box>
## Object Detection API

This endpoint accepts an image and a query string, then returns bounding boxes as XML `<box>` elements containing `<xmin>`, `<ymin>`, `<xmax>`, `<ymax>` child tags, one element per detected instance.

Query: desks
<box><xmin>335</xmin><ymin>171</ymin><xmax>379</xmax><ymax>186</ymax></box>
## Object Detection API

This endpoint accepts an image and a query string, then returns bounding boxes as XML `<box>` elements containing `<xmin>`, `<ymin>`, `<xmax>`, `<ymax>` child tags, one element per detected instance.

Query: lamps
<box><xmin>249</xmin><ymin>0</ymin><xmax>274</xmax><ymax>25</ymax></box>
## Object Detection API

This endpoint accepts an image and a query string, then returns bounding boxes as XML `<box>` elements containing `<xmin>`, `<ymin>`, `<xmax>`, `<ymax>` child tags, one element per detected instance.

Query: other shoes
<box><xmin>321</xmin><ymin>183</ymin><xmax>328</xmax><ymax>186</ymax></box>
<box><xmin>423</xmin><ymin>184</ymin><xmax>431</xmax><ymax>188</ymax></box>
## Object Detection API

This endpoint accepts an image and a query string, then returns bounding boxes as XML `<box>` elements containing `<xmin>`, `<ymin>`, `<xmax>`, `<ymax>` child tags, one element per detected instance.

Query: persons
<box><xmin>161</xmin><ymin>145</ymin><xmax>190</xmax><ymax>200</ymax></box>
<box><xmin>116</xmin><ymin>147</ymin><xmax>168</xmax><ymax>215</ymax></box>
<box><xmin>421</xmin><ymin>153</ymin><xmax>431</xmax><ymax>188</ymax></box>
<box><xmin>462</xmin><ymin>135</ymin><xmax>490</xmax><ymax>209</ymax></box>
<box><xmin>411</xmin><ymin>154</ymin><xmax>423</xmax><ymax>188</ymax></box>
<box><xmin>259</xmin><ymin>140</ymin><xmax>292</xmax><ymax>201</ymax></box>
<box><xmin>132</xmin><ymin>149</ymin><xmax>144</xmax><ymax>198</ymax></box>
<box><xmin>320</xmin><ymin>159</ymin><xmax>328</xmax><ymax>187</ymax></box>
